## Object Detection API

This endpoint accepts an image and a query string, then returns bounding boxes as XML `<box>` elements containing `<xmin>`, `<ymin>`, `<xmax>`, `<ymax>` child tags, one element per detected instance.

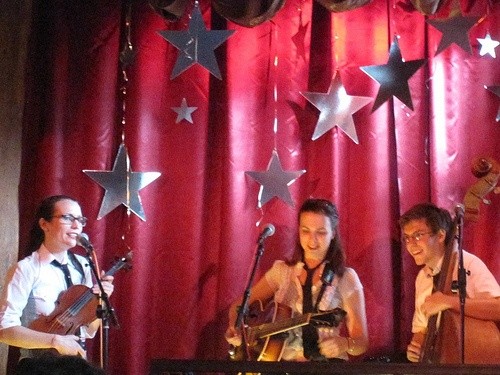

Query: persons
<box><xmin>1</xmin><ymin>196</ymin><xmax>115</xmax><ymax>361</ymax></box>
<box><xmin>225</xmin><ymin>199</ymin><xmax>368</xmax><ymax>364</ymax></box>
<box><xmin>11</xmin><ymin>355</ymin><xmax>105</xmax><ymax>375</ymax></box>
<box><xmin>398</xmin><ymin>203</ymin><xmax>500</xmax><ymax>364</ymax></box>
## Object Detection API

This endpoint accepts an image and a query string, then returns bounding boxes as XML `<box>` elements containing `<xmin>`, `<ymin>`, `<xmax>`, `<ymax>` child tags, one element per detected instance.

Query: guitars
<box><xmin>230</xmin><ymin>298</ymin><xmax>347</xmax><ymax>361</ymax></box>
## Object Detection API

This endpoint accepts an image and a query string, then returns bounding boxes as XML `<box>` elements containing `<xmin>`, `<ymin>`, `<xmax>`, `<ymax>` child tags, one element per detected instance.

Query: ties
<box><xmin>51</xmin><ymin>259</ymin><xmax>73</xmax><ymax>292</ymax></box>
<box><xmin>301</xmin><ymin>259</ymin><xmax>334</xmax><ymax>361</ymax></box>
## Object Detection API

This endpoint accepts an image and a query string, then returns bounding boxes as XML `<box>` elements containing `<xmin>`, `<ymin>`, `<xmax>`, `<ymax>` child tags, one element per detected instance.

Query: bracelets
<box><xmin>346</xmin><ymin>336</ymin><xmax>356</xmax><ymax>353</ymax></box>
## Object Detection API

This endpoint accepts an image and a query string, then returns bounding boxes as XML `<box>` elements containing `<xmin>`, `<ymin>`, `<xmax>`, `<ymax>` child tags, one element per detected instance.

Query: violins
<box><xmin>29</xmin><ymin>252</ymin><xmax>132</xmax><ymax>357</ymax></box>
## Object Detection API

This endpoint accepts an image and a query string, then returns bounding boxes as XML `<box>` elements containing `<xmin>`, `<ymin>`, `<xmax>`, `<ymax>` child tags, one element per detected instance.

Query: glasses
<box><xmin>401</xmin><ymin>228</ymin><xmax>439</xmax><ymax>243</ymax></box>
<box><xmin>49</xmin><ymin>213</ymin><xmax>89</xmax><ymax>227</ymax></box>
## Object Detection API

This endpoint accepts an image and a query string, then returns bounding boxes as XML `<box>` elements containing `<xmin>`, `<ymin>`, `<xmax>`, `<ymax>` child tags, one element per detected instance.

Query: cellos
<box><xmin>417</xmin><ymin>155</ymin><xmax>500</xmax><ymax>364</ymax></box>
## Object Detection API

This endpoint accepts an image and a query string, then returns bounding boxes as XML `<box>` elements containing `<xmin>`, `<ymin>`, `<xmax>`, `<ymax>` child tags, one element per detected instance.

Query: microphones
<box><xmin>453</xmin><ymin>201</ymin><xmax>465</xmax><ymax>218</ymax></box>
<box><xmin>76</xmin><ymin>233</ymin><xmax>93</xmax><ymax>252</ymax></box>
<box><xmin>257</xmin><ymin>223</ymin><xmax>275</xmax><ymax>243</ymax></box>
